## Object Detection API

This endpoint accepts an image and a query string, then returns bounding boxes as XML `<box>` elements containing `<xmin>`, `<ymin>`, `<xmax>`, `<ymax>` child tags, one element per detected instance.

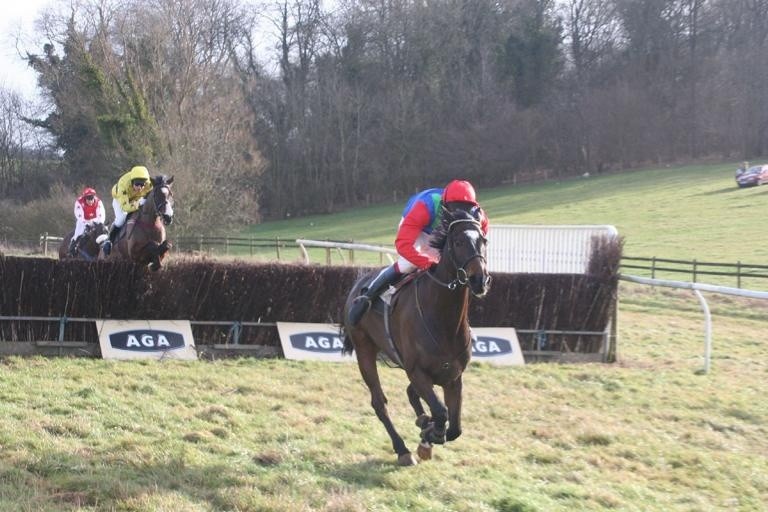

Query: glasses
<box><xmin>134</xmin><ymin>183</ymin><xmax>145</xmax><ymax>186</ymax></box>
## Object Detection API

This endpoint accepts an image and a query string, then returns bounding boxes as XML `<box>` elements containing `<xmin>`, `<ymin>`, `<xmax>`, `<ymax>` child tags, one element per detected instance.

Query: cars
<box><xmin>736</xmin><ymin>163</ymin><xmax>768</xmax><ymax>189</ymax></box>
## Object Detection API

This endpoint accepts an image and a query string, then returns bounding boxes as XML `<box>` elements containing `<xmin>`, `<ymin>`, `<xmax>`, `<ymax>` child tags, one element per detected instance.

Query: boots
<box><xmin>103</xmin><ymin>222</ymin><xmax>120</xmax><ymax>255</ymax></box>
<box><xmin>348</xmin><ymin>263</ymin><xmax>401</xmax><ymax>326</ymax></box>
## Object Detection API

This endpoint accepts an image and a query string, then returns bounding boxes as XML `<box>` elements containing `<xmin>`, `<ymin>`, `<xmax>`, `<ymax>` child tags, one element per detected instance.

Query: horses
<box><xmin>339</xmin><ymin>204</ymin><xmax>493</xmax><ymax>467</ymax></box>
<box><xmin>108</xmin><ymin>174</ymin><xmax>175</xmax><ymax>274</ymax></box>
<box><xmin>58</xmin><ymin>219</ymin><xmax>113</xmax><ymax>261</ymax></box>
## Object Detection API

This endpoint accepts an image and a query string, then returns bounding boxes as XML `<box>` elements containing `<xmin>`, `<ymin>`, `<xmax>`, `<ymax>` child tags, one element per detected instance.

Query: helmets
<box><xmin>130</xmin><ymin>166</ymin><xmax>149</xmax><ymax>181</ymax></box>
<box><xmin>84</xmin><ymin>188</ymin><xmax>96</xmax><ymax>196</ymax></box>
<box><xmin>443</xmin><ymin>180</ymin><xmax>478</xmax><ymax>206</ymax></box>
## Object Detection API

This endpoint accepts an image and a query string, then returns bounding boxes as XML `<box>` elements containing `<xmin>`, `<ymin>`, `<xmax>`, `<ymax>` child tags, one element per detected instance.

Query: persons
<box><xmin>349</xmin><ymin>180</ymin><xmax>488</xmax><ymax>327</ymax></box>
<box><xmin>103</xmin><ymin>166</ymin><xmax>153</xmax><ymax>255</ymax></box>
<box><xmin>68</xmin><ymin>188</ymin><xmax>105</xmax><ymax>251</ymax></box>
